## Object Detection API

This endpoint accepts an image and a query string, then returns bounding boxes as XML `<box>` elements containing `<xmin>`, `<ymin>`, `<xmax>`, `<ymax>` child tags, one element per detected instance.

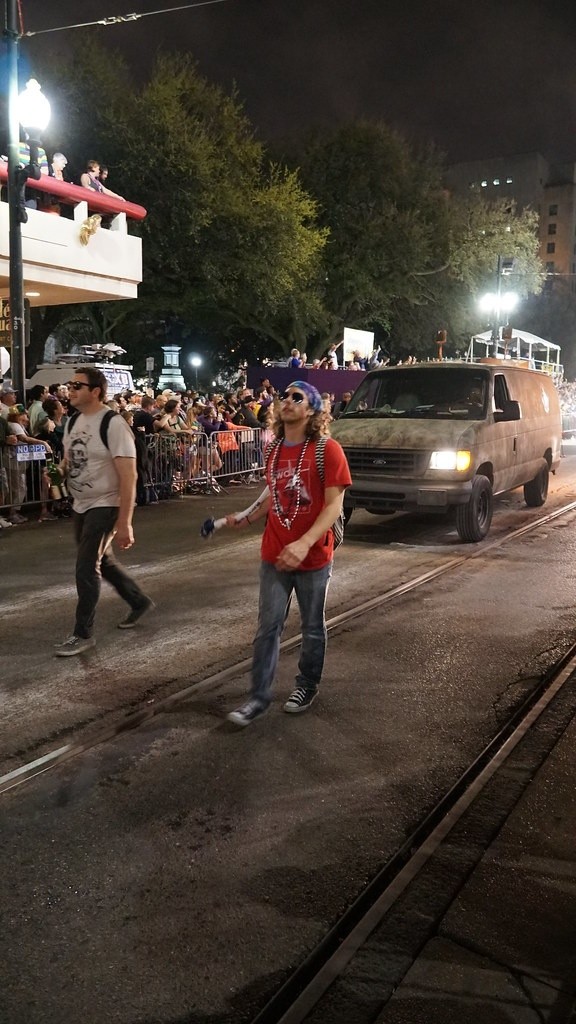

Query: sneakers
<box><xmin>40</xmin><ymin>508</ymin><xmax>71</xmax><ymax>520</ymax></box>
<box><xmin>118</xmin><ymin>595</ymin><xmax>156</xmax><ymax>627</ymax></box>
<box><xmin>53</xmin><ymin>633</ymin><xmax>96</xmax><ymax>656</ymax></box>
<box><xmin>283</xmin><ymin>685</ymin><xmax>320</xmax><ymax>713</ymax></box>
<box><xmin>226</xmin><ymin>695</ymin><xmax>272</xmax><ymax>725</ymax></box>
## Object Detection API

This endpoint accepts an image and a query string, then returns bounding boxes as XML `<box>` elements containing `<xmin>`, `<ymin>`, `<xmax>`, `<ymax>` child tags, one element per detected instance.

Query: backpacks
<box><xmin>265</xmin><ymin>435</ymin><xmax>344</xmax><ymax>551</ymax></box>
<box><xmin>68</xmin><ymin>410</ymin><xmax>148</xmax><ymax>506</ymax></box>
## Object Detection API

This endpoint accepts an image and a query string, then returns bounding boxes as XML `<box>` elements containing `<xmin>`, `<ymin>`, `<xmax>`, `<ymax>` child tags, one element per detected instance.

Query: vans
<box><xmin>25</xmin><ymin>341</ymin><xmax>136</xmax><ymax>402</ymax></box>
<box><xmin>323</xmin><ymin>356</ymin><xmax>564</xmax><ymax>543</ymax></box>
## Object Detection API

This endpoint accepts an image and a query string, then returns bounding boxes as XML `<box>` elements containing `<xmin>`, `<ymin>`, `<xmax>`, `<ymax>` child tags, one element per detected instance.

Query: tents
<box><xmin>470</xmin><ymin>327</ymin><xmax>562</xmax><ymax>372</ymax></box>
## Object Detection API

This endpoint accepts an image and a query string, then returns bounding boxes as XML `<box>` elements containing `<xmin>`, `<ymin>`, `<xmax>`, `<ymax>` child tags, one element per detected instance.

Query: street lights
<box><xmin>0</xmin><ymin>0</ymin><xmax>53</xmax><ymax>514</ymax></box>
<box><xmin>480</xmin><ymin>254</ymin><xmax>520</xmax><ymax>357</ymax></box>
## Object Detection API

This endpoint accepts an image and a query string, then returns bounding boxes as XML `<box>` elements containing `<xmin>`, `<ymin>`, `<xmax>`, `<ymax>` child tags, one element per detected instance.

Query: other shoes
<box><xmin>229</xmin><ymin>479</ymin><xmax>242</xmax><ymax>486</ymax></box>
<box><xmin>248</xmin><ymin>474</ymin><xmax>267</xmax><ymax>483</ymax></box>
<box><xmin>0</xmin><ymin>514</ymin><xmax>27</xmax><ymax>528</ymax></box>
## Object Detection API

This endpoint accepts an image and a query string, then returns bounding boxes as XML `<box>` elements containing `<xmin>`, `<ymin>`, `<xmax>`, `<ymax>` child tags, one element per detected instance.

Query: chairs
<box><xmin>394</xmin><ymin>393</ymin><xmax>422</xmax><ymax>410</ymax></box>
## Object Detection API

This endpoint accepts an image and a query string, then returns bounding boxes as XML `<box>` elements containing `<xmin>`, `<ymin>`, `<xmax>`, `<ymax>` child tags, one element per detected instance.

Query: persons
<box><xmin>286</xmin><ymin>340</ymin><xmax>344</xmax><ymax>370</ymax></box>
<box><xmin>464</xmin><ymin>378</ymin><xmax>504</xmax><ymax>413</ymax></box>
<box><xmin>348</xmin><ymin>344</ymin><xmax>390</xmax><ymax>370</ymax></box>
<box><xmin>104</xmin><ymin>379</ymin><xmax>277</xmax><ymax>507</ymax></box>
<box><xmin>321</xmin><ymin>389</ymin><xmax>355</xmax><ymax>419</ymax></box>
<box><xmin>224</xmin><ymin>381</ymin><xmax>353</xmax><ymax>727</ymax></box>
<box><xmin>42</xmin><ymin>367</ymin><xmax>154</xmax><ymax>656</ymax></box>
<box><xmin>0</xmin><ymin>381</ymin><xmax>76</xmax><ymax>528</ymax></box>
<box><xmin>19</xmin><ymin>130</ymin><xmax>126</xmax><ymax>230</ymax></box>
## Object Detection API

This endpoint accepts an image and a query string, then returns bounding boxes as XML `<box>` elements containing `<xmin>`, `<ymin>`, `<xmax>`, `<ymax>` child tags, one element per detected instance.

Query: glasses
<box><xmin>278</xmin><ymin>392</ymin><xmax>305</xmax><ymax>404</ymax></box>
<box><xmin>66</xmin><ymin>381</ymin><xmax>90</xmax><ymax>391</ymax></box>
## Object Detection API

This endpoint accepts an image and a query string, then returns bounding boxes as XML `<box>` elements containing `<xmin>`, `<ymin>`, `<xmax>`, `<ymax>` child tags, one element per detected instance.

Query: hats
<box><xmin>0</xmin><ymin>383</ymin><xmax>19</xmax><ymax>397</ymax></box>
<box><xmin>242</xmin><ymin>396</ymin><xmax>257</xmax><ymax>404</ymax></box>
<box><xmin>162</xmin><ymin>389</ymin><xmax>176</xmax><ymax>396</ymax></box>
<box><xmin>124</xmin><ymin>392</ymin><xmax>136</xmax><ymax>400</ymax></box>
<box><xmin>9</xmin><ymin>404</ymin><xmax>29</xmax><ymax>414</ymax></box>
<box><xmin>285</xmin><ymin>380</ymin><xmax>323</xmax><ymax>411</ymax></box>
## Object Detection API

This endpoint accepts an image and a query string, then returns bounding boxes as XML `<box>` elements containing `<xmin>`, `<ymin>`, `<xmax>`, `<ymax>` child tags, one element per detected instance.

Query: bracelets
<box><xmin>245</xmin><ymin>516</ymin><xmax>251</xmax><ymax>525</ymax></box>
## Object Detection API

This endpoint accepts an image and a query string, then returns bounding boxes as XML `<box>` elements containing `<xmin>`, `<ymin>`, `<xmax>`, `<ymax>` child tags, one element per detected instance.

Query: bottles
<box><xmin>356</xmin><ymin>398</ymin><xmax>368</xmax><ymax>411</ymax></box>
<box><xmin>45</xmin><ymin>452</ymin><xmax>68</xmax><ymax>500</ymax></box>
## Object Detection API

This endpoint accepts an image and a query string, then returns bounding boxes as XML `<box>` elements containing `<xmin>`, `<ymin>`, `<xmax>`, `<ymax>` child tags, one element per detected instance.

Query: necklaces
<box><xmin>270</xmin><ymin>437</ymin><xmax>309</xmax><ymax>528</ymax></box>
